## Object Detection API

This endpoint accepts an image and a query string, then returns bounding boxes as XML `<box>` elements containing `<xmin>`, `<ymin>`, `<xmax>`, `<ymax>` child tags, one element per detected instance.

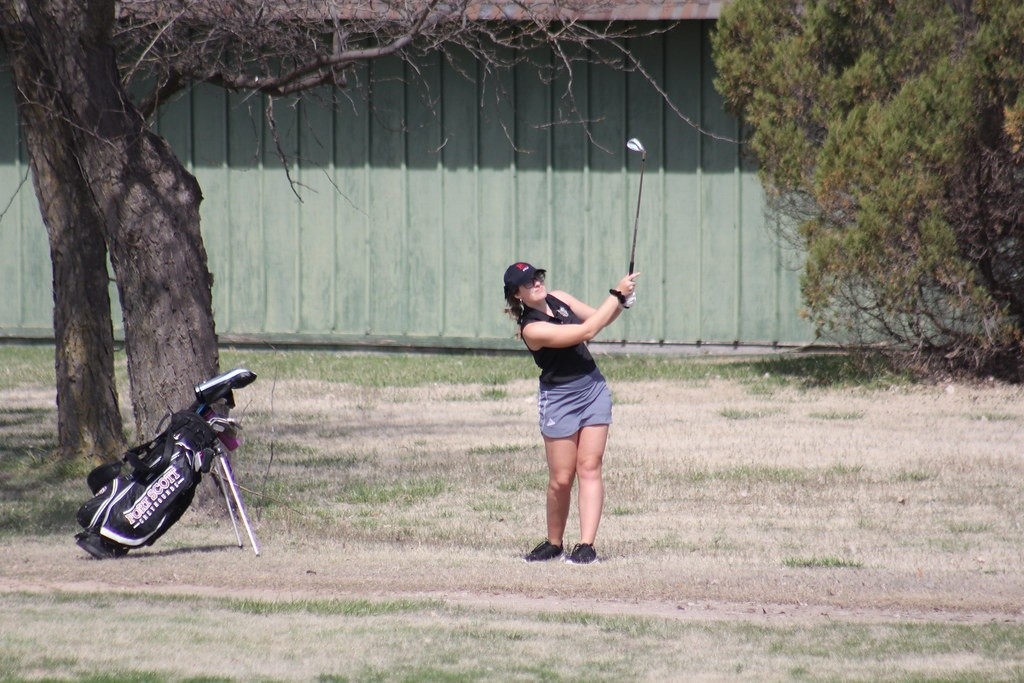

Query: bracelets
<box><xmin>609</xmin><ymin>289</ymin><xmax>625</xmax><ymax>304</ymax></box>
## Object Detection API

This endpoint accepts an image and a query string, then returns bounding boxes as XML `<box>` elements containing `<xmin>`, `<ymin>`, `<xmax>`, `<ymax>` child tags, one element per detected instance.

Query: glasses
<box><xmin>523</xmin><ymin>272</ymin><xmax>545</xmax><ymax>289</ymax></box>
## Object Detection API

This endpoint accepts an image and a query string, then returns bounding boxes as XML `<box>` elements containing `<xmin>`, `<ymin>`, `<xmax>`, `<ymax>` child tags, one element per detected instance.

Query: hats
<box><xmin>504</xmin><ymin>262</ymin><xmax>547</xmax><ymax>286</ymax></box>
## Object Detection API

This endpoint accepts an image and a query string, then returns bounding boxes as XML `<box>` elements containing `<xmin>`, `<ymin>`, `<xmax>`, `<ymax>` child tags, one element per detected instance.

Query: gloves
<box><xmin>618</xmin><ymin>291</ymin><xmax>636</xmax><ymax>310</ymax></box>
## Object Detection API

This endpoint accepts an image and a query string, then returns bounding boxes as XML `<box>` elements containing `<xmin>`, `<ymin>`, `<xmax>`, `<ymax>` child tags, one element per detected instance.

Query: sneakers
<box><xmin>566</xmin><ymin>543</ymin><xmax>599</xmax><ymax>566</ymax></box>
<box><xmin>523</xmin><ymin>540</ymin><xmax>563</xmax><ymax>563</ymax></box>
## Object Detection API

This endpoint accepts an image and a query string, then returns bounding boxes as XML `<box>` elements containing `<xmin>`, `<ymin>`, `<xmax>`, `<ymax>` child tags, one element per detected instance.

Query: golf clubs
<box><xmin>626</xmin><ymin>138</ymin><xmax>647</xmax><ymax>275</ymax></box>
<box><xmin>206</xmin><ymin>416</ymin><xmax>244</xmax><ymax>434</ymax></box>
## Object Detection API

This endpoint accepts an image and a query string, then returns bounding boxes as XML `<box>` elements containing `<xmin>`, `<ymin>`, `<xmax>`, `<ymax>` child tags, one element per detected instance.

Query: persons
<box><xmin>505</xmin><ymin>261</ymin><xmax>641</xmax><ymax>566</ymax></box>
